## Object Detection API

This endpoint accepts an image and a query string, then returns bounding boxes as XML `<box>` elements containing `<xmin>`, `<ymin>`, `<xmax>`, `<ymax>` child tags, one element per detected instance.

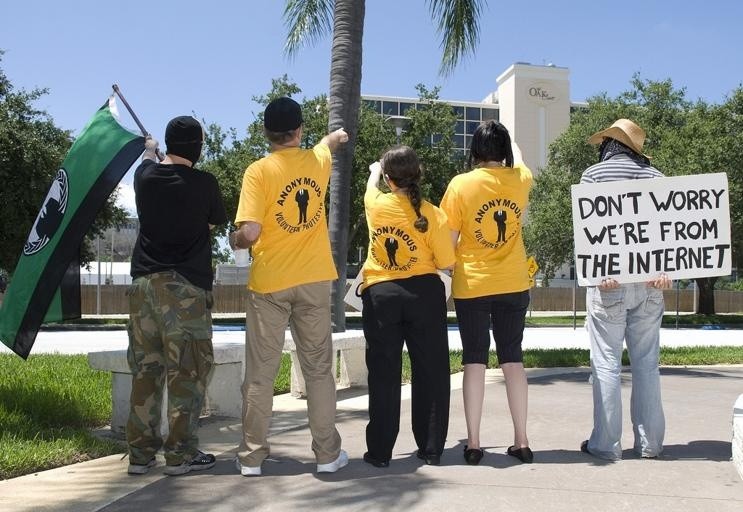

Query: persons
<box><xmin>579</xmin><ymin>119</ymin><xmax>673</xmax><ymax>462</ymax></box>
<box><xmin>440</xmin><ymin>118</ymin><xmax>535</xmax><ymax>464</ymax></box>
<box><xmin>362</xmin><ymin>145</ymin><xmax>456</xmax><ymax>466</ymax></box>
<box><xmin>124</xmin><ymin>116</ymin><xmax>228</xmax><ymax>478</ymax></box>
<box><xmin>229</xmin><ymin>96</ymin><xmax>350</xmax><ymax>478</ymax></box>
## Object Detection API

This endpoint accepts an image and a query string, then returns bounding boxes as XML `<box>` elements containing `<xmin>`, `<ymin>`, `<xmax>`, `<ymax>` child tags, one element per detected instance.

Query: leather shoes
<box><xmin>507</xmin><ymin>446</ymin><xmax>533</xmax><ymax>463</ymax></box>
<box><xmin>417</xmin><ymin>448</ymin><xmax>441</xmax><ymax>465</ymax></box>
<box><xmin>364</xmin><ymin>452</ymin><xmax>390</xmax><ymax>467</ymax></box>
<box><xmin>464</xmin><ymin>445</ymin><xmax>484</xmax><ymax>465</ymax></box>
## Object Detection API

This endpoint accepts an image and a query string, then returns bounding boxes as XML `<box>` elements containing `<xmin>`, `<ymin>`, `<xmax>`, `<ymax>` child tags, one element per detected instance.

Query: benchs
<box><xmin>282</xmin><ymin>329</ymin><xmax>367</xmax><ymax>397</ymax></box>
<box><xmin>88</xmin><ymin>342</ymin><xmax>245</xmax><ymax>437</ymax></box>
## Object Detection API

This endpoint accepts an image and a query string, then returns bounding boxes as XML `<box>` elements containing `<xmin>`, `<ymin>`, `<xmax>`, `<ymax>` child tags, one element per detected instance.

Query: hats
<box><xmin>588</xmin><ymin>118</ymin><xmax>654</xmax><ymax>160</ymax></box>
<box><xmin>264</xmin><ymin>96</ymin><xmax>305</xmax><ymax>131</ymax></box>
<box><xmin>166</xmin><ymin>116</ymin><xmax>203</xmax><ymax>163</ymax></box>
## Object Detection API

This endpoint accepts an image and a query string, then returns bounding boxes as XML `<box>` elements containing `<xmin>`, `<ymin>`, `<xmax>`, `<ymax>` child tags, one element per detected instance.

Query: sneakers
<box><xmin>163</xmin><ymin>449</ymin><xmax>216</xmax><ymax>476</ymax></box>
<box><xmin>581</xmin><ymin>440</ymin><xmax>588</xmax><ymax>454</ymax></box>
<box><xmin>234</xmin><ymin>456</ymin><xmax>262</xmax><ymax>476</ymax></box>
<box><xmin>128</xmin><ymin>456</ymin><xmax>156</xmax><ymax>474</ymax></box>
<box><xmin>317</xmin><ymin>449</ymin><xmax>348</xmax><ymax>473</ymax></box>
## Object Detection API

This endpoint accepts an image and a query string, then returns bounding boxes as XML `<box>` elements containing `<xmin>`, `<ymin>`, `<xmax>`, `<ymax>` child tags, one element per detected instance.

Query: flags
<box><xmin>0</xmin><ymin>96</ymin><xmax>148</xmax><ymax>362</ymax></box>
